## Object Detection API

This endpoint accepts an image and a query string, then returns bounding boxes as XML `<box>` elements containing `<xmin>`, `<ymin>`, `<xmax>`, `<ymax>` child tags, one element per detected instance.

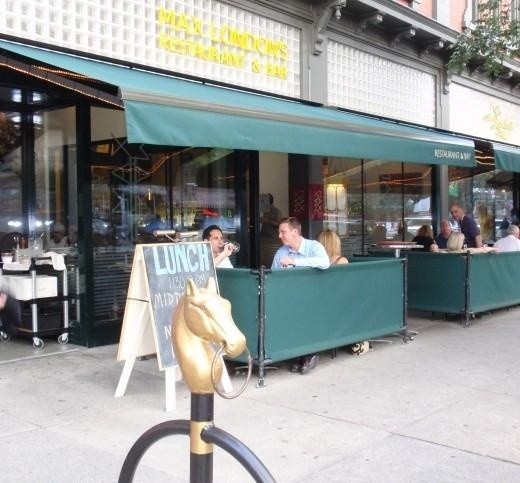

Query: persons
<box><xmin>492</xmin><ymin>223</ymin><xmax>520</xmax><ymax>252</ymax></box>
<box><xmin>449</xmin><ymin>200</ymin><xmax>482</xmax><ymax>248</ymax></box>
<box><xmin>269</xmin><ymin>215</ymin><xmax>330</xmax><ymax>375</ymax></box>
<box><xmin>261</xmin><ymin>192</ymin><xmax>282</xmax><ymax>269</ymax></box>
<box><xmin>201</xmin><ymin>223</ymin><xmax>237</xmax><ymax>269</ymax></box>
<box><xmin>45</xmin><ymin>211</ymin><xmax>234</xmax><ymax>250</ymax></box>
<box><xmin>410</xmin><ymin>224</ymin><xmax>439</xmax><ymax>251</ymax></box>
<box><xmin>314</xmin><ymin>226</ymin><xmax>373</xmax><ymax>355</ymax></box>
<box><xmin>436</xmin><ymin>218</ymin><xmax>455</xmax><ymax>248</ymax></box>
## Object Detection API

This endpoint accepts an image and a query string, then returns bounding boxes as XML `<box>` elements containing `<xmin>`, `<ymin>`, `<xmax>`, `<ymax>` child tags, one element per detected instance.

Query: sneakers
<box><xmin>351</xmin><ymin>341</ymin><xmax>369</xmax><ymax>357</ymax></box>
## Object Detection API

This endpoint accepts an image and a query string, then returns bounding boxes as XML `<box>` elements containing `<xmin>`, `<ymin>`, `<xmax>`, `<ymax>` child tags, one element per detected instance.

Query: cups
<box><xmin>0</xmin><ymin>253</ymin><xmax>13</xmax><ymax>263</ymax></box>
<box><xmin>28</xmin><ymin>238</ymin><xmax>43</xmax><ymax>258</ymax></box>
<box><xmin>220</xmin><ymin>239</ymin><xmax>240</xmax><ymax>252</ymax></box>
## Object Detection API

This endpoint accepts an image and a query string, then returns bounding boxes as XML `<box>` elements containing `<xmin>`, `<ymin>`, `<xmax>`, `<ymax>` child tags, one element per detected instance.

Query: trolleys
<box><xmin>0</xmin><ymin>254</ymin><xmax>68</xmax><ymax>348</ymax></box>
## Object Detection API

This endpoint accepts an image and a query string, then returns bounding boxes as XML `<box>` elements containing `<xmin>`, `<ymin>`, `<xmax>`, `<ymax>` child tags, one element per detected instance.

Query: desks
<box><xmin>370</xmin><ymin>240</ymin><xmax>425</xmax><ymax>258</ymax></box>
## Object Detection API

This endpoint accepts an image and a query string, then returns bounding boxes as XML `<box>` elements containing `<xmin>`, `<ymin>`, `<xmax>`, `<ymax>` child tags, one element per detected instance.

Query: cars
<box><xmin>194</xmin><ymin>209</ymin><xmax>241</xmax><ymax>230</ymax></box>
<box><xmin>323</xmin><ymin>199</ymin><xmax>462</xmax><ymax>236</ymax></box>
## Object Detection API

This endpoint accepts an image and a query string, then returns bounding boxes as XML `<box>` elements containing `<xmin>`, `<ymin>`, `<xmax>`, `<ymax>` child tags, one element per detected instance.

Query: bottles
<box><xmin>399</xmin><ymin>221</ymin><xmax>404</xmax><ymax>240</ymax></box>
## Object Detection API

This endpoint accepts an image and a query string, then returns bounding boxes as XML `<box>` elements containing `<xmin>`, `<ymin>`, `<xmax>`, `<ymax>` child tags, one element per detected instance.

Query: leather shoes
<box><xmin>291</xmin><ymin>353</ymin><xmax>319</xmax><ymax>374</ymax></box>
